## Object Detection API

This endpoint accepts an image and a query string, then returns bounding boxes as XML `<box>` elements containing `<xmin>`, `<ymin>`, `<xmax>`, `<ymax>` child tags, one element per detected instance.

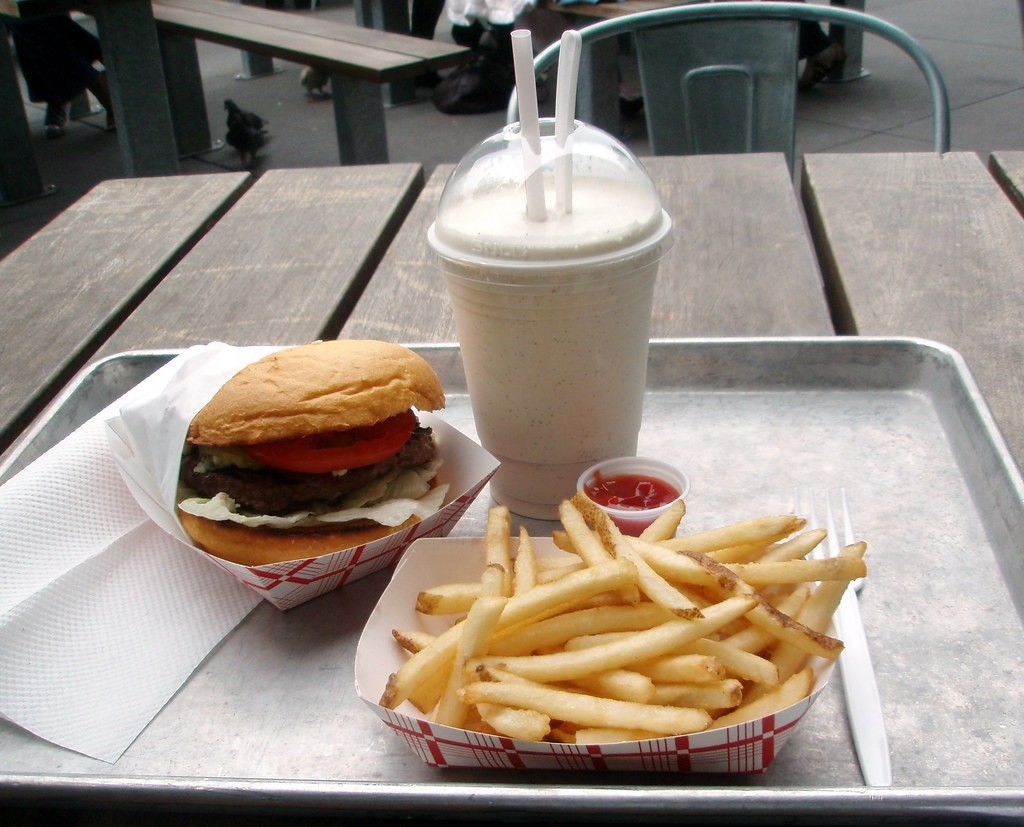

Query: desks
<box><xmin>351</xmin><ymin>537</ymin><xmax>836</xmax><ymax>773</ymax></box>
<box><xmin>0</xmin><ymin>151</ymin><xmax>1024</xmax><ymax>478</ymax></box>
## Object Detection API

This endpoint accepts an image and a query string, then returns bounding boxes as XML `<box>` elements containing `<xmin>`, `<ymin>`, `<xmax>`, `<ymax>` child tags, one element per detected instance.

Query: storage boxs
<box><xmin>106</xmin><ymin>382</ymin><xmax>502</xmax><ymax>611</ymax></box>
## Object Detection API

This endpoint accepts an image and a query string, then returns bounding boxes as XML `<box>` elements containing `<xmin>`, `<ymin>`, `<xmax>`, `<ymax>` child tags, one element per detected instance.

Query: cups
<box><xmin>578</xmin><ymin>457</ymin><xmax>688</xmax><ymax>538</ymax></box>
<box><xmin>428</xmin><ymin>118</ymin><xmax>674</xmax><ymax>519</ymax></box>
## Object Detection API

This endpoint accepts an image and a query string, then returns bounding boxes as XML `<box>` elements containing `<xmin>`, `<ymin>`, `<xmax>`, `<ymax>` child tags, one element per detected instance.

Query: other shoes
<box><xmin>70</xmin><ymin>95</ymin><xmax>98</xmax><ymax>121</ymax></box>
<box><xmin>43</xmin><ymin>104</ymin><xmax>67</xmax><ymax>141</ymax></box>
<box><xmin>799</xmin><ymin>42</ymin><xmax>848</xmax><ymax>92</ymax></box>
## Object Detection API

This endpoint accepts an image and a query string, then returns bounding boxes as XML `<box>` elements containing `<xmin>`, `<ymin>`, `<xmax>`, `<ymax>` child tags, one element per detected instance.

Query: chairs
<box><xmin>506</xmin><ymin>2</ymin><xmax>951</xmax><ymax>182</ymax></box>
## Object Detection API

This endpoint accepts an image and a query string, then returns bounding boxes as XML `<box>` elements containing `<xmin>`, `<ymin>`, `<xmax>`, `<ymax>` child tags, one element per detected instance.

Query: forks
<box><xmin>795</xmin><ymin>488</ymin><xmax>893</xmax><ymax>789</ymax></box>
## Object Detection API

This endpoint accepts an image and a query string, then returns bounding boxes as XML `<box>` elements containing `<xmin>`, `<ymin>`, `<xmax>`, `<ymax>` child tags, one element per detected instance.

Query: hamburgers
<box><xmin>178</xmin><ymin>338</ymin><xmax>445</xmax><ymax>566</ymax></box>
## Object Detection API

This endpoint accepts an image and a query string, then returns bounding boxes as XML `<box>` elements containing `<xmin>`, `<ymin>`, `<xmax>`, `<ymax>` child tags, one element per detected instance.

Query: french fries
<box><xmin>379</xmin><ymin>492</ymin><xmax>867</xmax><ymax>744</ymax></box>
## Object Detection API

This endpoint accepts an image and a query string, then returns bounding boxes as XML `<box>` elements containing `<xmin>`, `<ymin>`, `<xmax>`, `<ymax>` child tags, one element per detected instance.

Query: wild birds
<box><xmin>303</xmin><ymin>65</ymin><xmax>332</xmax><ymax>99</ymax></box>
<box><xmin>223</xmin><ymin>99</ymin><xmax>271</xmax><ymax>161</ymax></box>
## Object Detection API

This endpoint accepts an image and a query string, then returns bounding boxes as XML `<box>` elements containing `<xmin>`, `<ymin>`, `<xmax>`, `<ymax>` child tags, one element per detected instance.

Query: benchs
<box><xmin>545</xmin><ymin>0</ymin><xmax>702</xmax><ymax>110</ymax></box>
<box><xmin>61</xmin><ymin>0</ymin><xmax>471</xmax><ymax>167</ymax></box>
